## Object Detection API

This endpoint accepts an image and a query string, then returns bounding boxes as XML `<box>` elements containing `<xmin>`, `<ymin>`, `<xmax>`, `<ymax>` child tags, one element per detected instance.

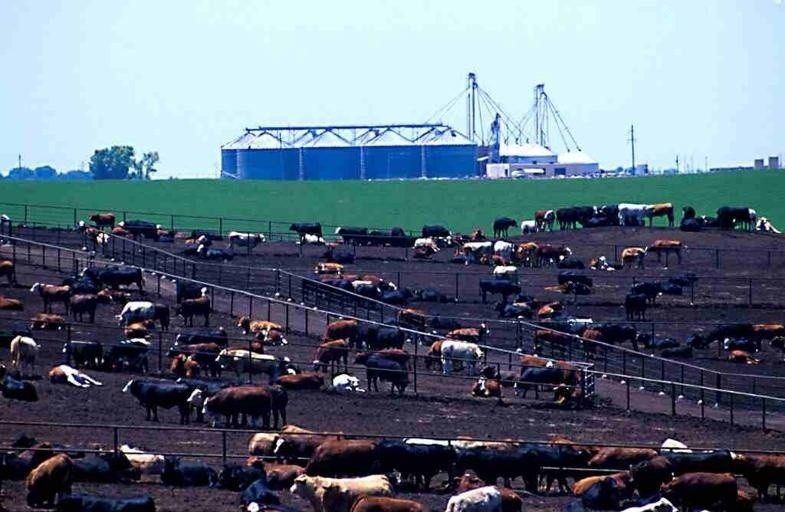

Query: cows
<box><xmin>0</xmin><ymin>201</ymin><xmax>785</xmax><ymax>511</ymax></box>
